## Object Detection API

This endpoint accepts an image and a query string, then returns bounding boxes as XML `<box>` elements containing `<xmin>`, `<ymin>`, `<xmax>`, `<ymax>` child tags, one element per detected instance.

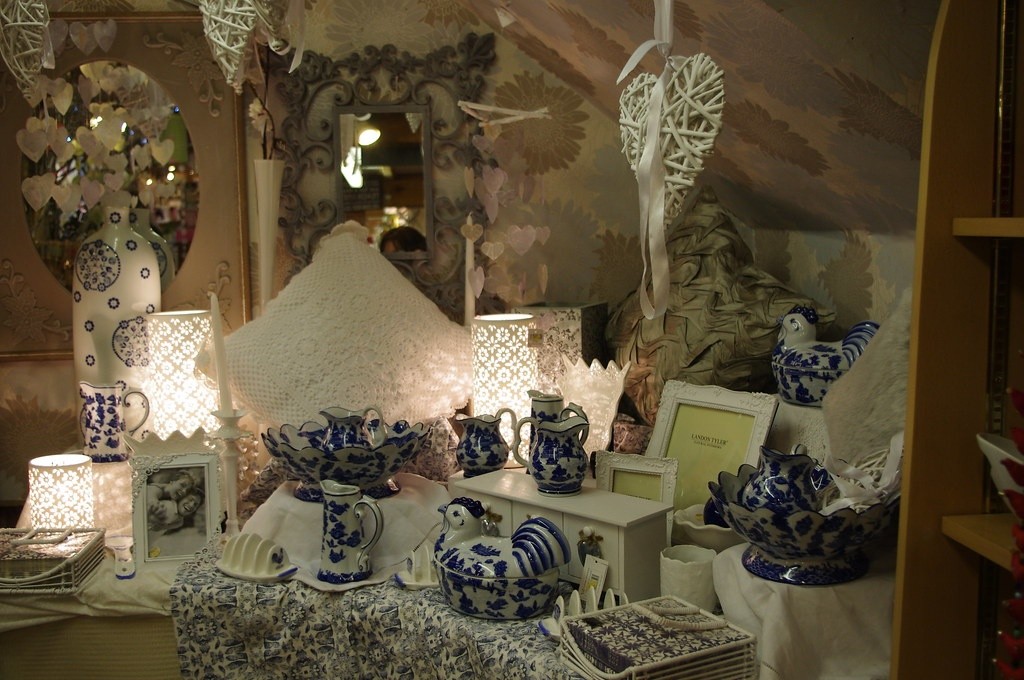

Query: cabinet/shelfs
<box><xmin>888</xmin><ymin>0</ymin><xmax>1024</xmax><ymax>679</ymax></box>
<box><xmin>456</xmin><ymin>470</ymin><xmax>674</xmax><ymax>602</ymax></box>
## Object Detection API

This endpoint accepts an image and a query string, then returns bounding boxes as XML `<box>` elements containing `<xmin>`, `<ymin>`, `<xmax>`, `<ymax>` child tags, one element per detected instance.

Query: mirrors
<box><xmin>1</xmin><ymin>11</ymin><xmax>252</xmax><ymax>362</ymax></box>
<box><xmin>334</xmin><ymin>102</ymin><xmax>433</xmax><ymax>260</ymax></box>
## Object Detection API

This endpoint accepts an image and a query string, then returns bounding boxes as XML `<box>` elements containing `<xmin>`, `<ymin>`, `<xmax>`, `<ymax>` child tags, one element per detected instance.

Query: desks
<box><xmin>166</xmin><ymin>535</ymin><xmax>614</xmax><ymax>680</ymax></box>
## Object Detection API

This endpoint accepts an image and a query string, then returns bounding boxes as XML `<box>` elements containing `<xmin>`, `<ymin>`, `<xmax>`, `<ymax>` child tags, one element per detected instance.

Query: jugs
<box><xmin>743</xmin><ymin>444</ymin><xmax>818</xmax><ymax>514</ymax></box>
<box><xmin>80</xmin><ymin>381</ymin><xmax>149</xmax><ymax>463</ymax></box>
<box><xmin>455</xmin><ymin>408</ymin><xmax>516</xmax><ymax>478</ymax></box>
<box><xmin>514</xmin><ymin>416</ymin><xmax>589</xmax><ymax>497</ymax></box>
<box><xmin>526</xmin><ymin>390</ymin><xmax>590</xmax><ymax>474</ymax></box>
<box><xmin>317</xmin><ymin>480</ymin><xmax>383</xmax><ymax>583</ymax></box>
<box><xmin>318</xmin><ymin>404</ymin><xmax>388</xmax><ymax>450</ymax></box>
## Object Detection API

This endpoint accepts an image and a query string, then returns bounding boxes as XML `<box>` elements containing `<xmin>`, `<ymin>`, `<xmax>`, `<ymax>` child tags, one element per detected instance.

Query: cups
<box><xmin>658</xmin><ymin>545</ymin><xmax>718</xmax><ymax>613</ymax></box>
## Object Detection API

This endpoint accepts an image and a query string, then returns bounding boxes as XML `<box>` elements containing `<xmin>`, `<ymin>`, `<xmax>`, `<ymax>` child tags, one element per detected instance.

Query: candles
<box><xmin>465</xmin><ymin>211</ymin><xmax>475</xmax><ymax>328</ymax></box>
<box><xmin>208</xmin><ymin>292</ymin><xmax>235</xmax><ymax>415</ymax></box>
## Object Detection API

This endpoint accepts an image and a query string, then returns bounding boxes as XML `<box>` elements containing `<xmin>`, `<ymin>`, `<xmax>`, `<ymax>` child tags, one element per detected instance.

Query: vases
<box><xmin>74</xmin><ymin>206</ymin><xmax>162</xmax><ymax>462</ymax></box>
<box><xmin>130</xmin><ymin>207</ymin><xmax>176</xmax><ymax>295</ymax></box>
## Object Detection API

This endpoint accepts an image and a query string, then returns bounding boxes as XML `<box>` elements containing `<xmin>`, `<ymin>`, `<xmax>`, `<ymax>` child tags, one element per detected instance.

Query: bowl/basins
<box><xmin>673</xmin><ymin>504</ymin><xmax>748</xmax><ymax>555</ymax></box>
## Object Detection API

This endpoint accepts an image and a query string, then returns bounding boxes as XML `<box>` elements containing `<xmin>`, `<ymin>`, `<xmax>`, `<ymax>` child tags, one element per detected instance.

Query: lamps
<box><xmin>355</xmin><ymin>121</ymin><xmax>380</xmax><ymax>145</ymax></box>
<box><xmin>147</xmin><ymin>307</ymin><xmax>216</xmax><ymax>434</ymax></box>
<box><xmin>471</xmin><ymin>313</ymin><xmax>540</xmax><ymax>415</ymax></box>
<box><xmin>30</xmin><ymin>456</ymin><xmax>93</xmax><ymax>529</ymax></box>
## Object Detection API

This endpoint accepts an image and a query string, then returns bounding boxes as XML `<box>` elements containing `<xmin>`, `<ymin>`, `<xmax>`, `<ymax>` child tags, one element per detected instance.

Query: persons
<box><xmin>380</xmin><ymin>225</ymin><xmax>428</xmax><ymax>252</ymax></box>
<box><xmin>147</xmin><ymin>487</ymin><xmax>205</xmax><ymax>534</ymax></box>
<box><xmin>147</xmin><ymin>470</ymin><xmax>194</xmax><ymax>519</ymax></box>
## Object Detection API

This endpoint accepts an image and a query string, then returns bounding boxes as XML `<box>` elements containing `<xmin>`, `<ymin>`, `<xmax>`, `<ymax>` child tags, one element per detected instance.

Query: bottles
<box><xmin>73</xmin><ymin>208</ymin><xmax>161</xmax><ymax>450</ymax></box>
<box><xmin>107</xmin><ymin>536</ymin><xmax>136</xmax><ymax>579</ymax></box>
<box><xmin>129</xmin><ymin>208</ymin><xmax>174</xmax><ymax>293</ymax></box>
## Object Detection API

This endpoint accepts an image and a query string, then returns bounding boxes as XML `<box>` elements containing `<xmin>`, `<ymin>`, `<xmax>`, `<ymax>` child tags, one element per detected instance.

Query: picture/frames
<box><xmin>645</xmin><ymin>380</ymin><xmax>779</xmax><ymax>509</ymax></box>
<box><xmin>593</xmin><ymin>450</ymin><xmax>679</xmax><ymax>548</ymax></box>
<box><xmin>127</xmin><ymin>451</ymin><xmax>223</xmax><ymax>562</ymax></box>
